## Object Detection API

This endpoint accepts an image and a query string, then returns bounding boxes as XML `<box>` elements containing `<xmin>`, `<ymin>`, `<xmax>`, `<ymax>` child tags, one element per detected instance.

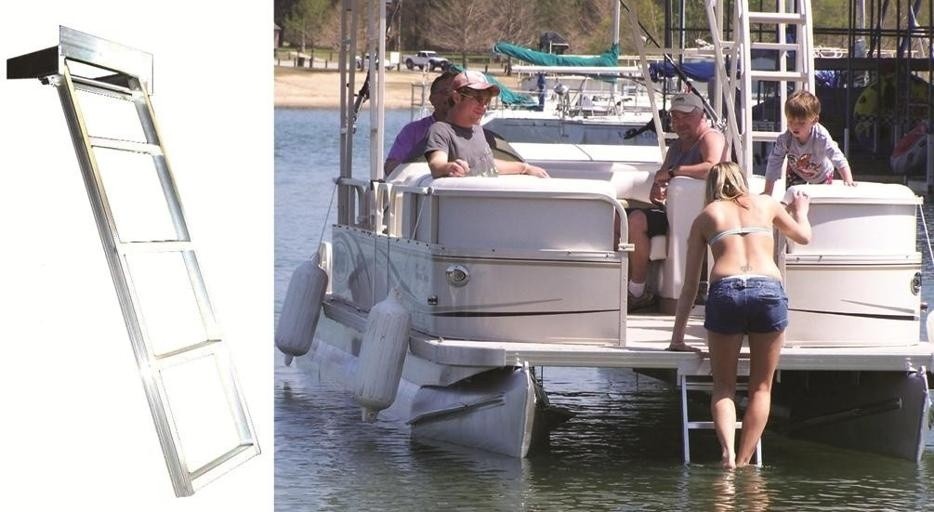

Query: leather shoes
<box><xmin>629</xmin><ymin>288</ymin><xmax>655</xmax><ymax>308</ymax></box>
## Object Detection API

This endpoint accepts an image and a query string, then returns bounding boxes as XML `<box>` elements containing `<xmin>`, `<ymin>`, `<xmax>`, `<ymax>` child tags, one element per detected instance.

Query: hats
<box><xmin>672</xmin><ymin>93</ymin><xmax>705</xmax><ymax>114</ymax></box>
<box><xmin>452</xmin><ymin>69</ymin><xmax>500</xmax><ymax>96</ymax></box>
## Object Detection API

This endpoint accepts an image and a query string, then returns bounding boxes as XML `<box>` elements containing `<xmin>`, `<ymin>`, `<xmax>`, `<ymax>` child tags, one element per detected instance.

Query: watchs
<box><xmin>668</xmin><ymin>165</ymin><xmax>674</xmax><ymax>178</ymax></box>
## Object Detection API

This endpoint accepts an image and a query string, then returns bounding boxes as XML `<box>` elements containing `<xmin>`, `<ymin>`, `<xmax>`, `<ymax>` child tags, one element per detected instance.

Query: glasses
<box><xmin>461</xmin><ymin>92</ymin><xmax>492</xmax><ymax>106</ymax></box>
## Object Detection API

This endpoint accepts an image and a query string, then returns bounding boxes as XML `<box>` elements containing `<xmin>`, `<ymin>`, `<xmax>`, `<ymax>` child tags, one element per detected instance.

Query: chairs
<box><xmin>655</xmin><ymin>174</ymin><xmax>707</xmax><ymax>300</ymax></box>
<box><xmin>385</xmin><ymin>162</ymin><xmax>434</xmax><ymax>246</ymax></box>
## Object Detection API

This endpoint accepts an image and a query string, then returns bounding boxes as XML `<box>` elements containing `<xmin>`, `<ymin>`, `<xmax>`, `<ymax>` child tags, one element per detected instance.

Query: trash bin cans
<box><xmin>298</xmin><ymin>57</ymin><xmax>305</xmax><ymax>67</ymax></box>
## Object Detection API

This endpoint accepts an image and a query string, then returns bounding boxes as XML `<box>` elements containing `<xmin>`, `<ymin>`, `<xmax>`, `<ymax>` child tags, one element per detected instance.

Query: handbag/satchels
<box><xmin>650</xmin><ymin>182</ymin><xmax>669</xmax><ymax>205</ymax></box>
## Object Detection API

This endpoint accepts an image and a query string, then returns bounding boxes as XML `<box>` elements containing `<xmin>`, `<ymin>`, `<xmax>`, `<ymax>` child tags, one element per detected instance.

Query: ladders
<box><xmin>5</xmin><ymin>26</ymin><xmax>263</xmax><ymax>498</ymax></box>
<box><xmin>724</xmin><ymin>0</ymin><xmax>817</xmax><ymax>192</ymax></box>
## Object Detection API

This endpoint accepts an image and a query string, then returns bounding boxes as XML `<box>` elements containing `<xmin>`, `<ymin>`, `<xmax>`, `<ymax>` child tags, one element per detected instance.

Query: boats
<box><xmin>275</xmin><ymin>0</ymin><xmax>934</xmax><ymax>462</ymax></box>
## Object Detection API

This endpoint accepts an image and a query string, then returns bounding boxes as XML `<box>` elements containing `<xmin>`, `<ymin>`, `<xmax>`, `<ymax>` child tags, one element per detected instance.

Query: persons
<box><xmin>670</xmin><ymin>161</ymin><xmax>811</xmax><ymax>469</ymax></box>
<box><xmin>384</xmin><ymin>68</ymin><xmax>551</xmax><ymax>181</ymax></box>
<box><xmin>761</xmin><ymin>90</ymin><xmax>856</xmax><ymax>196</ymax></box>
<box><xmin>615</xmin><ymin>92</ymin><xmax>726</xmax><ymax>313</ymax></box>
